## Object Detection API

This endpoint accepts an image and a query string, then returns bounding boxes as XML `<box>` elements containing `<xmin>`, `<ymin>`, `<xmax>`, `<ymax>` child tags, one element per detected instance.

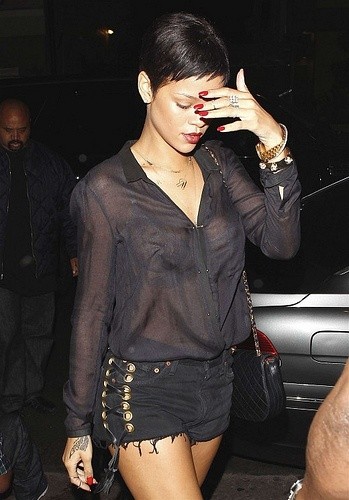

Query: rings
<box><xmin>76</xmin><ymin>480</ymin><xmax>82</xmax><ymax>489</ymax></box>
<box><xmin>68</xmin><ymin>476</ymin><xmax>79</xmax><ymax>479</ymax></box>
<box><xmin>229</xmin><ymin>94</ymin><xmax>239</xmax><ymax>108</ymax></box>
<box><xmin>212</xmin><ymin>99</ymin><xmax>215</xmax><ymax>110</ymax></box>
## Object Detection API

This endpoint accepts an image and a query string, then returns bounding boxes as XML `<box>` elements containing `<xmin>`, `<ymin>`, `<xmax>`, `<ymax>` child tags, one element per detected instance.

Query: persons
<box><xmin>58</xmin><ymin>16</ymin><xmax>301</xmax><ymax>500</ymax></box>
<box><xmin>0</xmin><ymin>409</ymin><xmax>49</xmax><ymax>500</ymax></box>
<box><xmin>287</xmin><ymin>357</ymin><xmax>349</xmax><ymax>500</ymax></box>
<box><xmin>0</xmin><ymin>96</ymin><xmax>77</xmax><ymax>419</ymax></box>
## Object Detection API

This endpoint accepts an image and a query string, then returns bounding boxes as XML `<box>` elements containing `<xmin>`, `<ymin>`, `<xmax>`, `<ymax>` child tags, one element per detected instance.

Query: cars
<box><xmin>244</xmin><ymin>167</ymin><xmax>349</xmax><ymax>469</ymax></box>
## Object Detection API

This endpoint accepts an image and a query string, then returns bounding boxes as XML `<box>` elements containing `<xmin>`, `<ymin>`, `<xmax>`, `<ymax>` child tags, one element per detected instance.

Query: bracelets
<box><xmin>255</xmin><ymin>124</ymin><xmax>293</xmax><ymax>171</ymax></box>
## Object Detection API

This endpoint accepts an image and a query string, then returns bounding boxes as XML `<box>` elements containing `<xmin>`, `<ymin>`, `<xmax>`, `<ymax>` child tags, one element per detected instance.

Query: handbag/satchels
<box><xmin>231</xmin><ymin>328</ymin><xmax>287</xmax><ymax>423</ymax></box>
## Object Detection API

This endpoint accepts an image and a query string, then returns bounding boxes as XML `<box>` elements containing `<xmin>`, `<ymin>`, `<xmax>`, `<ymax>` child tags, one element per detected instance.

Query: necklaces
<box><xmin>132</xmin><ymin>148</ymin><xmax>192</xmax><ymax>190</ymax></box>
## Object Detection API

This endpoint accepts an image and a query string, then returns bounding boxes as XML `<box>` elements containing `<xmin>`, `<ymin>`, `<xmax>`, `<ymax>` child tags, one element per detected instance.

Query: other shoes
<box><xmin>7</xmin><ymin>409</ymin><xmax>24</xmax><ymax>418</ymax></box>
<box><xmin>16</xmin><ymin>474</ymin><xmax>48</xmax><ymax>500</ymax></box>
<box><xmin>26</xmin><ymin>396</ymin><xmax>54</xmax><ymax>412</ymax></box>
